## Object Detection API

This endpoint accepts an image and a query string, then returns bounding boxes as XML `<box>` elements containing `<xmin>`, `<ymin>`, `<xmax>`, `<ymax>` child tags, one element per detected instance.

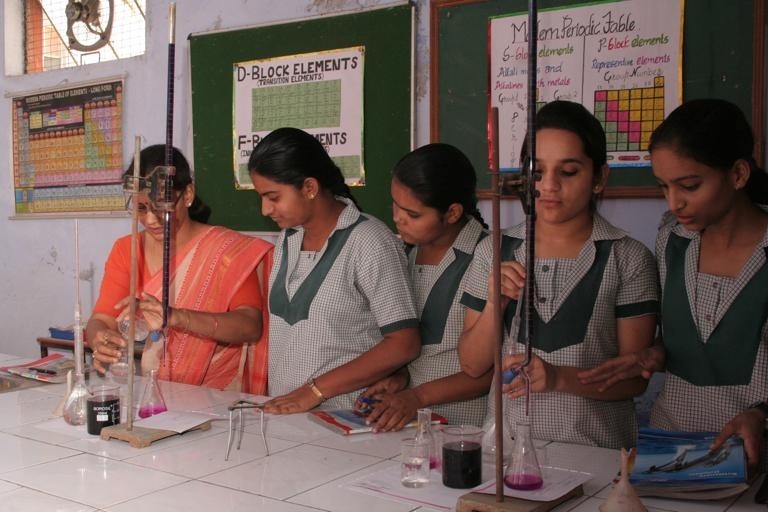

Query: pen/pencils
<box><xmin>356</xmin><ymin>397</ymin><xmax>379</xmax><ymax>404</ymax></box>
<box><xmin>29</xmin><ymin>367</ymin><xmax>56</xmax><ymax>375</ymax></box>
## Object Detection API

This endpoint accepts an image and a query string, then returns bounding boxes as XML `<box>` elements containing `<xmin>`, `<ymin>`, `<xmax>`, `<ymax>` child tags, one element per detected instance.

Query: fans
<box><xmin>66</xmin><ymin>0</ymin><xmax>113</xmax><ymax>51</ymax></box>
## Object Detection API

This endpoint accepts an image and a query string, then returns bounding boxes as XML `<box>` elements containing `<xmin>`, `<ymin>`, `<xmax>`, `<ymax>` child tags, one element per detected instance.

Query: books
<box><xmin>307</xmin><ymin>408</ymin><xmax>448</xmax><ymax>437</ymax></box>
<box><xmin>629</xmin><ymin>425</ymin><xmax>750</xmax><ymax>501</ymax></box>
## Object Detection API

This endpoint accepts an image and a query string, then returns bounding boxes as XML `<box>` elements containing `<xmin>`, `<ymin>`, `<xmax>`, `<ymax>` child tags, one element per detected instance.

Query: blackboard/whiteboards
<box><xmin>430</xmin><ymin>1</ymin><xmax>767</xmax><ymax>200</ymax></box>
<box><xmin>187</xmin><ymin>1</ymin><xmax>417</xmax><ymax>236</ymax></box>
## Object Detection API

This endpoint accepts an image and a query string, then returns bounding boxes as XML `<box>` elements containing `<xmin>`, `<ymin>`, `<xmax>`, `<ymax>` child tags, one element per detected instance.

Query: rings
<box><xmin>104</xmin><ymin>332</ymin><xmax>111</xmax><ymax>346</ymax></box>
<box><xmin>92</xmin><ymin>351</ymin><xmax>97</xmax><ymax>359</ymax></box>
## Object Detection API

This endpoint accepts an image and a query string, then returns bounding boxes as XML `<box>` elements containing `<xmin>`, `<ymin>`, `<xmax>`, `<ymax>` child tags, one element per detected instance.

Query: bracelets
<box><xmin>183</xmin><ymin>308</ymin><xmax>190</xmax><ymax>333</ymax></box>
<box><xmin>199</xmin><ymin>312</ymin><xmax>218</xmax><ymax>339</ymax></box>
<box><xmin>308</xmin><ymin>378</ymin><xmax>323</xmax><ymax>399</ymax></box>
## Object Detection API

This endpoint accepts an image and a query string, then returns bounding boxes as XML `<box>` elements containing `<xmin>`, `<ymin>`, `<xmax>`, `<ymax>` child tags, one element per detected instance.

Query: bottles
<box><xmin>414</xmin><ymin>407</ymin><xmax>441</xmax><ymax>468</ymax></box>
<box><xmin>139</xmin><ymin>368</ymin><xmax>168</xmax><ymax>416</ymax></box>
<box><xmin>484</xmin><ymin>400</ymin><xmax>549</xmax><ymax>487</ymax></box>
<box><xmin>62</xmin><ymin>372</ymin><xmax>96</xmax><ymax>428</ymax></box>
<box><xmin>99</xmin><ymin>315</ymin><xmax>149</xmax><ymax>383</ymax></box>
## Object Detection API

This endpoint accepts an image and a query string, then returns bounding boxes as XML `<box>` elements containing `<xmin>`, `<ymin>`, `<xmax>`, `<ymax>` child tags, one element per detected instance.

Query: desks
<box><xmin>35</xmin><ymin>337</ymin><xmax>146</xmax><ymax>370</ymax></box>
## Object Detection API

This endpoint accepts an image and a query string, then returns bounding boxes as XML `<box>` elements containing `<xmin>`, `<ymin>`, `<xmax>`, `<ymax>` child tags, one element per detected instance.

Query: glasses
<box><xmin>124</xmin><ymin>191</ymin><xmax>186</xmax><ymax>217</ymax></box>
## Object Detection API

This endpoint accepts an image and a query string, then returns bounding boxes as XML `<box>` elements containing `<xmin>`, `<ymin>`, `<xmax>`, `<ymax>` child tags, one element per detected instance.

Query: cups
<box><xmin>441</xmin><ymin>428</ymin><xmax>484</xmax><ymax>488</ymax></box>
<box><xmin>402</xmin><ymin>441</ymin><xmax>430</xmax><ymax>489</ymax></box>
<box><xmin>87</xmin><ymin>386</ymin><xmax>120</xmax><ymax>434</ymax></box>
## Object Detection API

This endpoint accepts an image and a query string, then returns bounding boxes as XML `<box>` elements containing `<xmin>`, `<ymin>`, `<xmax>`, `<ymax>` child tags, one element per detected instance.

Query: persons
<box><xmin>353</xmin><ymin>141</ymin><xmax>498</xmax><ymax>428</ymax></box>
<box><xmin>458</xmin><ymin>101</ymin><xmax>661</xmax><ymax>451</ymax></box>
<box><xmin>85</xmin><ymin>144</ymin><xmax>275</xmax><ymax>396</ymax></box>
<box><xmin>575</xmin><ymin>95</ymin><xmax>768</xmax><ymax>466</ymax></box>
<box><xmin>247</xmin><ymin>127</ymin><xmax>422</xmax><ymax>415</ymax></box>
